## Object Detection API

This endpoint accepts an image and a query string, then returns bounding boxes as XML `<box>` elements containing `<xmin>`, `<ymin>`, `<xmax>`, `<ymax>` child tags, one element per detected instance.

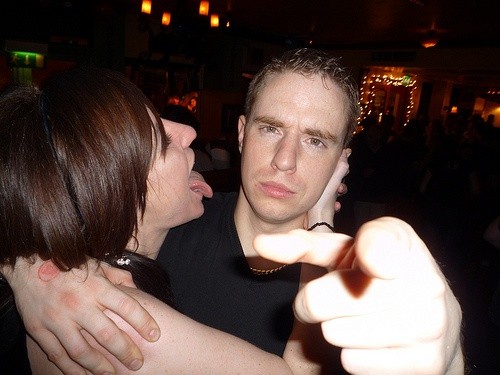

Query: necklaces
<box><xmin>251</xmin><ymin>265</ymin><xmax>289</xmax><ymax>278</ymax></box>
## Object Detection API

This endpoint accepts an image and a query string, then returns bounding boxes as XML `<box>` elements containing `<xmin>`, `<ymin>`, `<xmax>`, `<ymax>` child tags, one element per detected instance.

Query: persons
<box><xmin>1</xmin><ymin>46</ymin><xmax>491</xmax><ymax>375</ymax></box>
<box><xmin>0</xmin><ymin>69</ymin><xmax>351</xmax><ymax>375</ymax></box>
<box><xmin>344</xmin><ymin>104</ymin><xmax>500</xmax><ymax>242</ymax></box>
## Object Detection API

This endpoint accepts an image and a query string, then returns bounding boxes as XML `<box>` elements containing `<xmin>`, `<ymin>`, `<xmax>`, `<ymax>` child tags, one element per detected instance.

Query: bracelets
<box><xmin>308</xmin><ymin>222</ymin><xmax>334</xmax><ymax>236</ymax></box>
<box><xmin>99</xmin><ymin>248</ymin><xmax>171</xmax><ymax>306</ymax></box>
